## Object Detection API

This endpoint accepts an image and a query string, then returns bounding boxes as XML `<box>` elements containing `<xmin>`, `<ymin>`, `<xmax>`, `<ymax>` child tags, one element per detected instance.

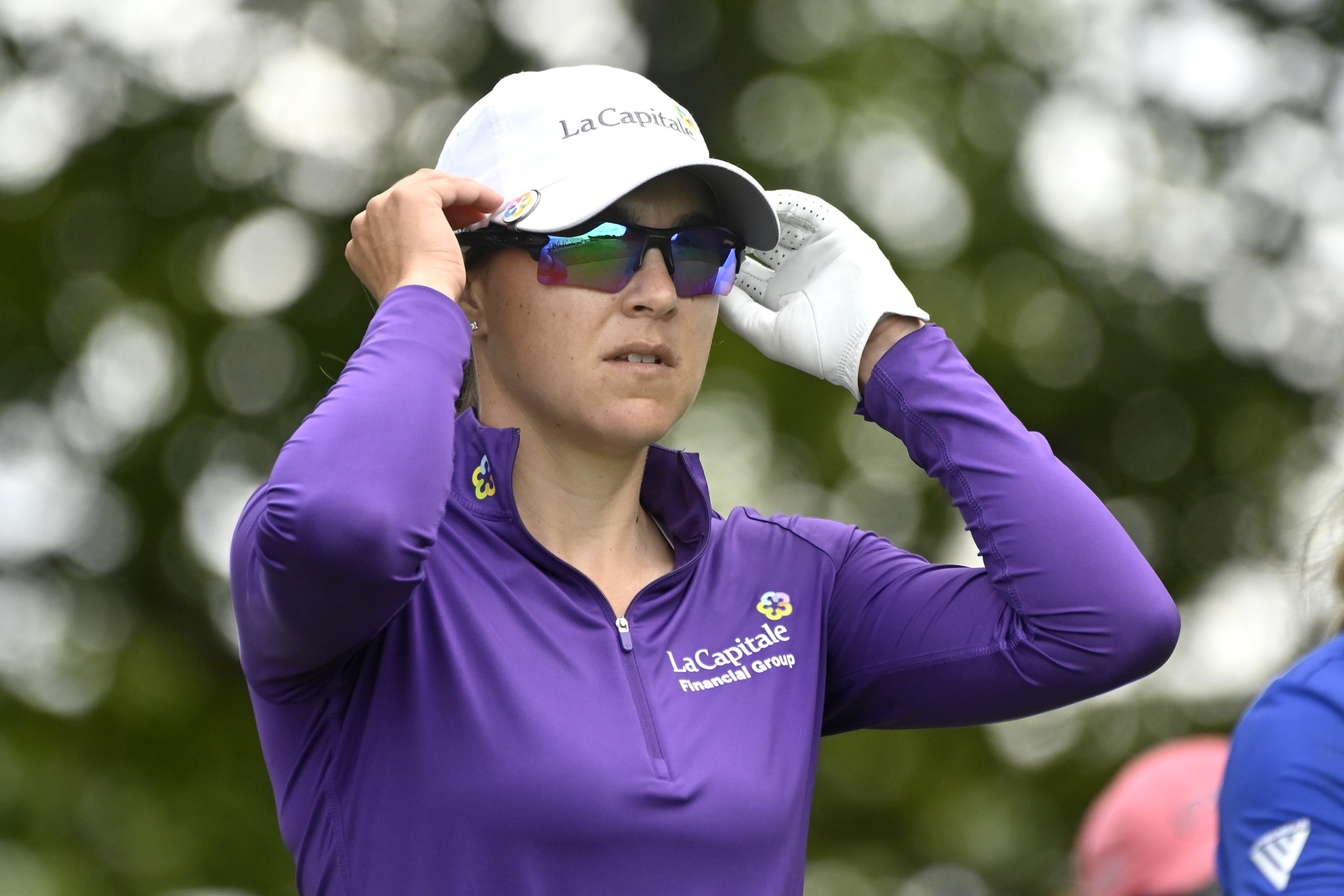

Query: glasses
<box><xmin>456</xmin><ymin>220</ymin><xmax>747</xmax><ymax>299</ymax></box>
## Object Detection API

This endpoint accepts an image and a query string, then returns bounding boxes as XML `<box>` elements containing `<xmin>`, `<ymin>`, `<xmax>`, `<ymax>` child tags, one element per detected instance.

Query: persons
<box><xmin>226</xmin><ymin>61</ymin><xmax>1184</xmax><ymax>896</ymax></box>
<box><xmin>1070</xmin><ymin>547</ymin><xmax>1344</xmax><ymax>896</ymax></box>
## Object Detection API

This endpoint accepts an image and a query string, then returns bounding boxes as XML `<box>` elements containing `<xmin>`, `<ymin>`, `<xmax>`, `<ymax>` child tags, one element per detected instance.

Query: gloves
<box><xmin>718</xmin><ymin>189</ymin><xmax>930</xmax><ymax>403</ymax></box>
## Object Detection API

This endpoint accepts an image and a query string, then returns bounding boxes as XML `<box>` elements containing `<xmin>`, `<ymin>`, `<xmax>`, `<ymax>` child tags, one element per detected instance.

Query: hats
<box><xmin>434</xmin><ymin>65</ymin><xmax>780</xmax><ymax>252</ymax></box>
